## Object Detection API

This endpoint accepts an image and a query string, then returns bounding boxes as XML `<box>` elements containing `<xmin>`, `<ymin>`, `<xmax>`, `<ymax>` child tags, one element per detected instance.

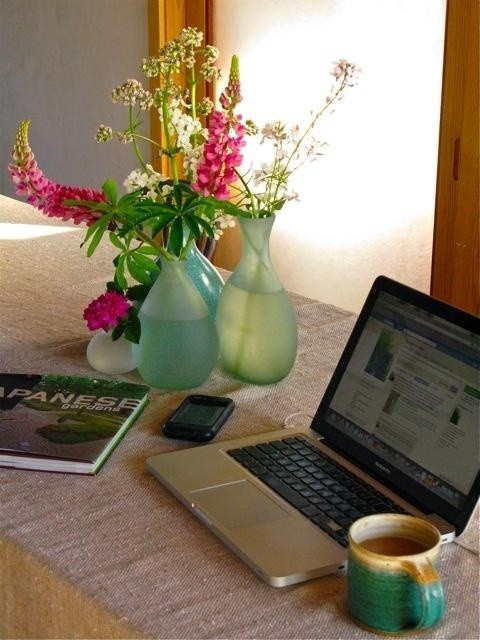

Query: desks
<box><xmin>1</xmin><ymin>194</ymin><xmax>478</xmax><ymax>638</ymax></box>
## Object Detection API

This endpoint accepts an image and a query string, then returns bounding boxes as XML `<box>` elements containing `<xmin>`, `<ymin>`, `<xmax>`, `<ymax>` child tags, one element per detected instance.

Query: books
<box><xmin>1</xmin><ymin>373</ymin><xmax>151</xmax><ymax>476</ymax></box>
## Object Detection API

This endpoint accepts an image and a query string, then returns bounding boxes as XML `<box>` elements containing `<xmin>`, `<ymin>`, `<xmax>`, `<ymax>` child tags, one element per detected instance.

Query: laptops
<box><xmin>146</xmin><ymin>275</ymin><xmax>480</xmax><ymax>588</ymax></box>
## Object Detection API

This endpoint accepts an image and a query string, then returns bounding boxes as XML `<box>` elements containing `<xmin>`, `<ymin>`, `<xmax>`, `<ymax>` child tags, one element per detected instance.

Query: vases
<box><xmin>216</xmin><ymin>207</ymin><xmax>298</xmax><ymax>385</ymax></box>
<box><xmin>83</xmin><ymin>319</ymin><xmax>140</xmax><ymax>376</ymax></box>
<box><xmin>131</xmin><ymin>253</ymin><xmax>221</xmax><ymax>393</ymax></box>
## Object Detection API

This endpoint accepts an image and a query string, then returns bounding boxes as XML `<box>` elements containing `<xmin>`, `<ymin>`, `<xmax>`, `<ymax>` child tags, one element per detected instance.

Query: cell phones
<box><xmin>162</xmin><ymin>395</ymin><xmax>235</xmax><ymax>442</ymax></box>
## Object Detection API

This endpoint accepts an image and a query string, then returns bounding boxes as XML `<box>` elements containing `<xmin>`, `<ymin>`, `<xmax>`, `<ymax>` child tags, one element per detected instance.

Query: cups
<box><xmin>345</xmin><ymin>513</ymin><xmax>446</xmax><ymax>637</ymax></box>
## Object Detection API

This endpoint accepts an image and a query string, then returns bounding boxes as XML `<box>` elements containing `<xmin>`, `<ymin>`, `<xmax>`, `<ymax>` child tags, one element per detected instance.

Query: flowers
<box><xmin>6</xmin><ymin>27</ymin><xmax>361</xmax><ymax>349</ymax></box>
